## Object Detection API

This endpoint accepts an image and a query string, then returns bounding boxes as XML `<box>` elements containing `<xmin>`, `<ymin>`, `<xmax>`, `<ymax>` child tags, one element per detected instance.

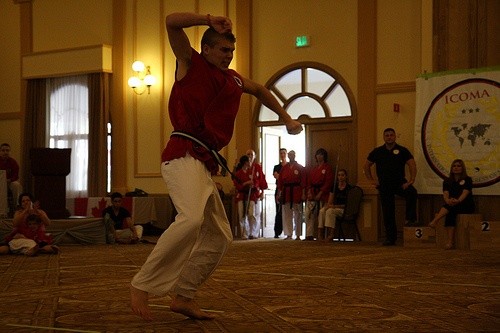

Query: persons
<box><xmin>315</xmin><ymin>168</ymin><xmax>352</xmax><ymax>241</ymax></box>
<box><xmin>303</xmin><ymin>147</ymin><xmax>333</xmax><ymax>241</ymax></box>
<box><xmin>275</xmin><ymin>151</ymin><xmax>307</xmax><ymax>241</ymax></box>
<box><xmin>427</xmin><ymin>159</ymin><xmax>476</xmax><ymax>249</ymax></box>
<box><xmin>231</xmin><ymin>155</ymin><xmax>261</xmax><ymax>239</ymax></box>
<box><xmin>245</xmin><ymin>149</ymin><xmax>268</xmax><ymax>239</ymax></box>
<box><xmin>363</xmin><ymin>127</ymin><xmax>421</xmax><ymax>246</ymax></box>
<box><xmin>273</xmin><ymin>148</ymin><xmax>290</xmax><ymax>239</ymax></box>
<box><xmin>101</xmin><ymin>192</ymin><xmax>143</xmax><ymax>244</ymax></box>
<box><xmin>0</xmin><ymin>143</ymin><xmax>60</xmax><ymax>257</ymax></box>
<box><xmin>129</xmin><ymin>12</ymin><xmax>303</xmax><ymax>320</ymax></box>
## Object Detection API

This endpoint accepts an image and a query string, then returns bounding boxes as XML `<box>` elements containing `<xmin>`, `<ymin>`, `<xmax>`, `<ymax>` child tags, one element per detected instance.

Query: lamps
<box><xmin>127</xmin><ymin>61</ymin><xmax>156</xmax><ymax>95</ymax></box>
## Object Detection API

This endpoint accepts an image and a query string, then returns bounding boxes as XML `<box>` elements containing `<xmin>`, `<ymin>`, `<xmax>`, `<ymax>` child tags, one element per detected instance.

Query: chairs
<box><xmin>335</xmin><ymin>186</ymin><xmax>363</xmax><ymax>242</ymax></box>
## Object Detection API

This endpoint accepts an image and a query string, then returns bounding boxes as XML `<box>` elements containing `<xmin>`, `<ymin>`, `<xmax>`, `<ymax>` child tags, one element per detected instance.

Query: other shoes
<box><xmin>404</xmin><ymin>220</ymin><xmax>420</xmax><ymax>227</ymax></box>
<box><xmin>382</xmin><ymin>240</ymin><xmax>396</xmax><ymax>246</ymax></box>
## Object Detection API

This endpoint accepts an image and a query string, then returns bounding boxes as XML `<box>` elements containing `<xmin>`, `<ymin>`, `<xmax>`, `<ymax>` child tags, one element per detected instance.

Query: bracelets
<box><xmin>207</xmin><ymin>13</ymin><xmax>212</xmax><ymax>27</ymax></box>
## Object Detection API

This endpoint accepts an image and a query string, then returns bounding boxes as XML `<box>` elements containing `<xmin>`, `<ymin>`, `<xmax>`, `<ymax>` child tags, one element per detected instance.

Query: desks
<box><xmin>74</xmin><ymin>197</ymin><xmax>173</xmax><ymax>236</ymax></box>
